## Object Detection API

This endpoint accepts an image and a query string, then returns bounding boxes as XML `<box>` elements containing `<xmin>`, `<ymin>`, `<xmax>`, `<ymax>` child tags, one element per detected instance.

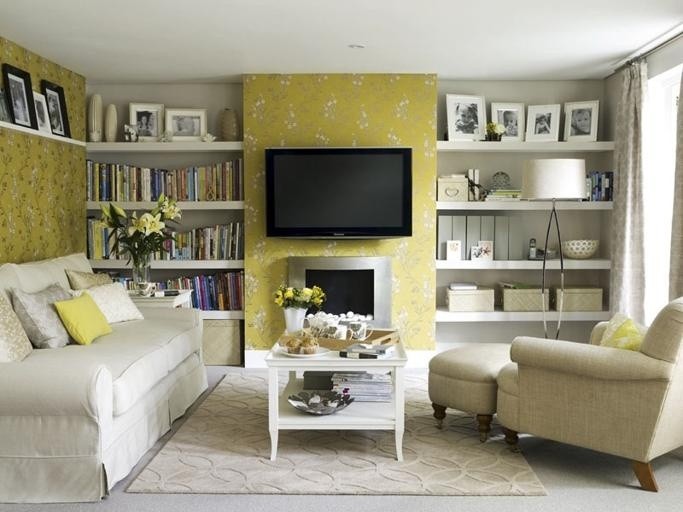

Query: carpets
<box><xmin>123</xmin><ymin>373</ymin><xmax>548</xmax><ymax>496</ymax></box>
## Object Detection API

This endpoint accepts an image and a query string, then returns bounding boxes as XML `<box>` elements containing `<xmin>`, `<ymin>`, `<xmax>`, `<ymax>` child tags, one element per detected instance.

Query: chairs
<box><xmin>493</xmin><ymin>297</ymin><xmax>682</xmax><ymax>492</ymax></box>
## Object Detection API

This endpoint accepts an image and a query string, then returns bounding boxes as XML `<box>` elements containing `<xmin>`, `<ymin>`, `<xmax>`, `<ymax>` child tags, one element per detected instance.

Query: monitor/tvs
<box><xmin>264</xmin><ymin>147</ymin><xmax>413</xmax><ymax>237</ymax></box>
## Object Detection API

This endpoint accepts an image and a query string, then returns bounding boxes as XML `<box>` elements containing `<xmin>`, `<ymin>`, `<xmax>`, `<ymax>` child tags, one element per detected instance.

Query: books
<box><xmin>300</xmin><ymin>374</ymin><xmax>393</xmax><ymax>403</ymax></box>
<box><xmin>449</xmin><ymin>280</ymin><xmax>478</xmax><ymax>289</ymax></box>
<box><xmin>466</xmin><ymin>168</ymin><xmax>524</xmax><ymax>201</ymax></box>
<box><xmin>80</xmin><ymin>159</ymin><xmax>244</xmax><ymax>312</ymax></box>
<box><xmin>338</xmin><ymin>343</ymin><xmax>394</xmax><ymax>360</ymax></box>
<box><xmin>585</xmin><ymin>169</ymin><xmax>613</xmax><ymax>204</ymax></box>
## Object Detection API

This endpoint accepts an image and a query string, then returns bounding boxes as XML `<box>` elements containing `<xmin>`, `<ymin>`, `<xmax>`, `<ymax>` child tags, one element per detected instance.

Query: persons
<box><xmin>570</xmin><ymin>109</ymin><xmax>591</xmax><ymax>136</ymax></box>
<box><xmin>455</xmin><ymin>104</ymin><xmax>481</xmax><ymax>134</ymax></box>
<box><xmin>135</xmin><ymin>109</ymin><xmax>158</xmax><ymax>136</ymax></box>
<box><xmin>535</xmin><ymin>112</ymin><xmax>551</xmax><ymax>136</ymax></box>
<box><xmin>497</xmin><ymin>110</ymin><xmax>520</xmax><ymax>137</ymax></box>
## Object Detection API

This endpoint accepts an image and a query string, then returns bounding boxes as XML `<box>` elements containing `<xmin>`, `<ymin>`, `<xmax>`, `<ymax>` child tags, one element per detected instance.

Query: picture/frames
<box><xmin>443</xmin><ymin>92</ymin><xmax>600</xmax><ymax>143</ymax></box>
<box><xmin>128</xmin><ymin>100</ymin><xmax>208</xmax><ymax>141</ymax></box>
<box><xmin>0</xmin><ymin>60</ymin><xmax>71</xmax><ymax>140</ymax></box>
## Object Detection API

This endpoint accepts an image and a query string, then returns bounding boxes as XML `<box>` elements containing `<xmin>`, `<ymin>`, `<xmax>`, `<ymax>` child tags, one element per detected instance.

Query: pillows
<box><xmin>600</xmin><ymin>312</ymin><xmax>650</xmax><ymax>351</ymax></box>
<box><xmin>0</xmin><ymin>267</ymin><xmax>142</xmax><ymax>366</ymax></box>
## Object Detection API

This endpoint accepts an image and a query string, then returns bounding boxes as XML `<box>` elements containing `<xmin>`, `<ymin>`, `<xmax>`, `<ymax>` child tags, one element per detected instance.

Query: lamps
<box><xmin>519</xmin><ymin>159</ymin><xmax>587</xmax><ymax>339</ymax></box>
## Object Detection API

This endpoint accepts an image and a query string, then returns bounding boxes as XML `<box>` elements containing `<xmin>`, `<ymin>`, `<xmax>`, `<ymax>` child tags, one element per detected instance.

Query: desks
<box><xmin>262</xmin><ymin>327</ymin><xmax>408</xmax><ymax>464</ymax></box>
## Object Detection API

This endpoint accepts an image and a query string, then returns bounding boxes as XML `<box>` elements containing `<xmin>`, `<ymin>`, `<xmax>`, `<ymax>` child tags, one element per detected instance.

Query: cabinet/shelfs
<box><xmin>436</xmin><ymin>139</ymin><xmax>616</xmax><ymax>323</ymax></box>
<box><xmin>84</xmin><ymin>139</ymin><xmax>243</xmax><ymax>320</ymax></box>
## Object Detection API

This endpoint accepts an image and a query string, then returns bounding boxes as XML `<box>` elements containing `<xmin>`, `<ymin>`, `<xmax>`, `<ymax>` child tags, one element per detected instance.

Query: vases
<box><xmin>282</xmin><ymin>304</ymin><xmax>309</xmax><ymax>333</ymax></box>
<box><xmin>131</xmin><ymin>253</ymin><xmax>154</xmax><ymax>297</ymax></box>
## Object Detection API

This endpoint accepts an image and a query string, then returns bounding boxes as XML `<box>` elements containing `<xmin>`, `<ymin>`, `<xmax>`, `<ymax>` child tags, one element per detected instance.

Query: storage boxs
<box><xmin>445</xmin><ymin>285</ymin><xmax>607</xmax><ymax>313</ymax></box>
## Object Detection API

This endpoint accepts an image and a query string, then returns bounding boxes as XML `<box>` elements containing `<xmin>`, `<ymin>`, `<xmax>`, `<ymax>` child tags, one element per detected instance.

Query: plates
<box><xmin>280</xmin><ymin>348</ymin><xmax>329</xmax><ymax>357</ymax></box>
<box><xmin>289</xmin><ymin>391</ymin><xmax>353</xmax><ymax>414</ymax></box>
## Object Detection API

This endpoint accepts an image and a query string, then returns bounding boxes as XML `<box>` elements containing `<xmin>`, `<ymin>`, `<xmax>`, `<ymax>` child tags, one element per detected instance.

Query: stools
<box><xmin>425</xmin><ymin>342</ymin><xmax>512</xmax><ymax>444</ymax></box>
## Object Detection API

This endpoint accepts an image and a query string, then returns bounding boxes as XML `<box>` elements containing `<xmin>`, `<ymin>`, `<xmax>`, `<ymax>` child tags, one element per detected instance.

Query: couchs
<box><xmin>0</xmin><ymin>249</ymin><xmax>204</xmax><ymax>506</ymax></box>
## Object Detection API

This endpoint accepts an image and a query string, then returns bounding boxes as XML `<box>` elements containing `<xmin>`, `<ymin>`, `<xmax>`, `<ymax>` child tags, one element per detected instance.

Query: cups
<box><xmin>349</xmin><ymin>321</ymin><xmax>373</xmax><ymax>340</ymax></box>
<box><xmin>327</xmin><ymin>324</ymin><xmax>353</xmax><ymax>340</ymax></box>
<box><xmin>137</xmin><ymin>283</ymin><xmax>157</xmax><ymax>297</ymax></box>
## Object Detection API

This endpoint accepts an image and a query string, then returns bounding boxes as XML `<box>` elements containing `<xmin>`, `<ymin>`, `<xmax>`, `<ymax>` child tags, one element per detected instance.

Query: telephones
<box><xmin>528</xmin><ymin>238</ymin><xmax>544</xmax><ymax>260</ymax></box>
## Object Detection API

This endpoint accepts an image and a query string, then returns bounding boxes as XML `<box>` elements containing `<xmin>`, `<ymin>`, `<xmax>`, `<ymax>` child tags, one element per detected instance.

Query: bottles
<box><xmin>220</xmin><ymin>107</ymin><xmax>241</xmax><ymax>141</ymax></box>
<box><xmin>529</xmin><ymin>238</ymin><xmax>536</xmax><ymax>259</ymax></box>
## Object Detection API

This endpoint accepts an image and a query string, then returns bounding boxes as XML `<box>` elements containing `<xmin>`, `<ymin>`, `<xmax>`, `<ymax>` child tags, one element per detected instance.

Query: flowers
<box><xmin>96</xmin><ymin>196</ymin><xmax>182</xmax><ymax>282</ymax></box>
<box><xmin>272</xmin><ymin>283</ymin><xmax>325</xmax><ymax>310</ymax></box>
